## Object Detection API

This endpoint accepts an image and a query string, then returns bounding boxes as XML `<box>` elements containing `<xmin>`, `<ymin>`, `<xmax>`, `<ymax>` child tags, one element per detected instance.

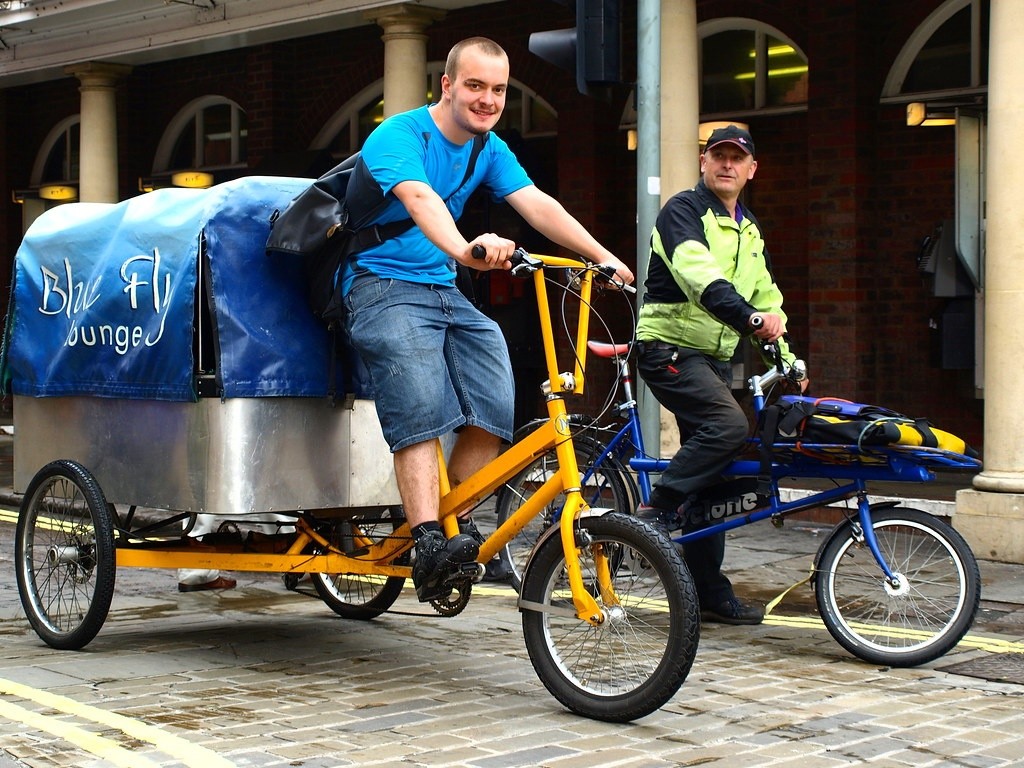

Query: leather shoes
<box><xmin>178</xmin><ymin>575</ymin><xmax>236</xmax><ymax>592</ymax></box>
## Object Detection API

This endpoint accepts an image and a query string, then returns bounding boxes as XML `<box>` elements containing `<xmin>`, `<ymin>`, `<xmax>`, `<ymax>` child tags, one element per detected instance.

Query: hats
<box><xmin>703</xmin><ymin>125</ymin><xmax>756</xmax><ymax>162</ymax></box>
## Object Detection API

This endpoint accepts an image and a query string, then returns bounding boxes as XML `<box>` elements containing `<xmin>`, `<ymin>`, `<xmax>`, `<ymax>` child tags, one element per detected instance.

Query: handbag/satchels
<box><xmin>264</xmin><ymin>150</ymin><xmax>361</xmax><ymax>274</ymax></box>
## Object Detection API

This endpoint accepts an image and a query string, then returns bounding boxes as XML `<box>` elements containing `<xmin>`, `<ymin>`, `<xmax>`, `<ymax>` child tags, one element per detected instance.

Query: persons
<box><xmin>177</xmin><ymin>511</ymin><xmax>326</xmax><ymax>593</ymax></box>
<box><xmin>633</xmin><ymin>124</ymin><xmax>808</xmax><ymax>624</ymax></box>
<box><xmin>330</xmin><ymin>38</ymin><xmax>632</xmax><ymax>602</ymax></box>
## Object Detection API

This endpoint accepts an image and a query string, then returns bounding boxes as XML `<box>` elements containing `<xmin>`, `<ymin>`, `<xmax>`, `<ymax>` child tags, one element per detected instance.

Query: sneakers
<box><xmin>700</xmin><ymin>598</ymin><xmax>764</xmax><ymax>624</ymax></box>
<box><xmin>412</xmin><ymin>525</ymin><xmax>479</xmax><ymax>603</ymax></box>
<box><xmin>458</xmin><ymin>518</ymin><xmax>514</xmax><ymax>583</ymax></box>
<box><xmin>633</xmin><ymin>503</ymin><xmax>684</xmax><ymax>554</ymax></box>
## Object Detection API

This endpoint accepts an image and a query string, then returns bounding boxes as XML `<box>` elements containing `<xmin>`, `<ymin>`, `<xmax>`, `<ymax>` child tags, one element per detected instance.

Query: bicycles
<box><xmin>493</xmin><ymin>316</ymin><xmax>986</xmax><ymax>669</ymax></box>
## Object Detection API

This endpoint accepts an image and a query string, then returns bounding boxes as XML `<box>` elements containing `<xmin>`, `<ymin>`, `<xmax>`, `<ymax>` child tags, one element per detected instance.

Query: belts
<box><xmin>645</xmin><ymin>341</ymin><xmax>675</xmax><ymax>350</ymax></box>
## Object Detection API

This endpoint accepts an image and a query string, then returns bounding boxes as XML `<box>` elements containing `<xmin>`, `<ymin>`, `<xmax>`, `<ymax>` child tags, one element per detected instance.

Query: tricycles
<box><xmin>0</xmin><ymin>179</ymin><xmax>706</xmax><ymax>723</ymax></box>
